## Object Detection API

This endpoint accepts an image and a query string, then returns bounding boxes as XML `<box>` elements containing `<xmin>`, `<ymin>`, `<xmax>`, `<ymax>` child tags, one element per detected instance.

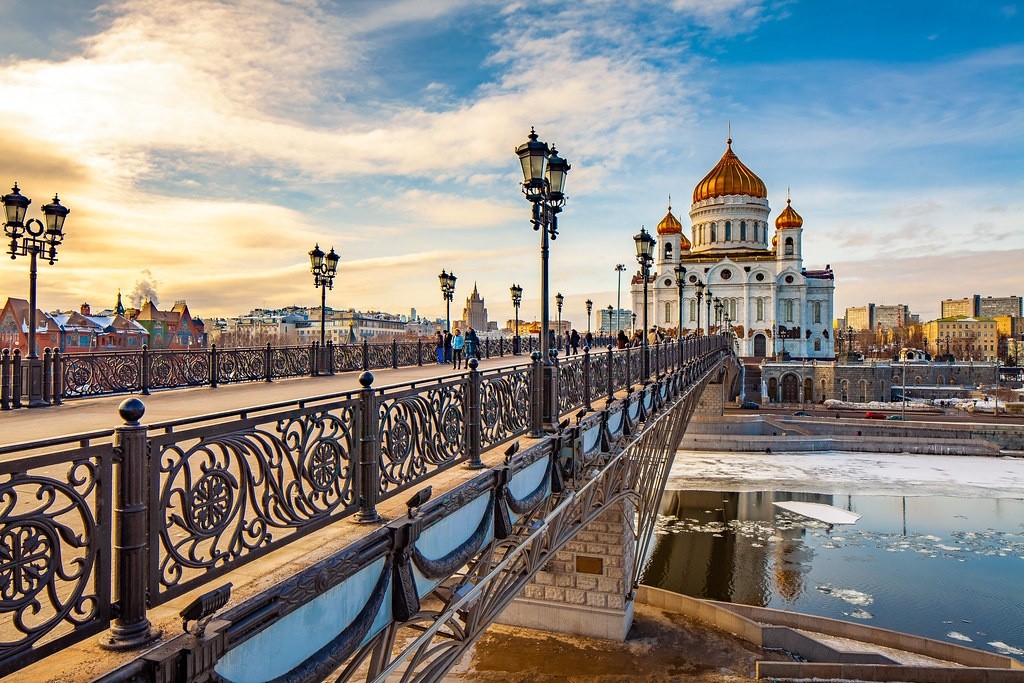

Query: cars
<box><xmin>741</xmin><ymin>402</ymin><xmax>759</xmax><ymax>409</ymax></box>
<box><xmin>863</xmin><ymin>411</ymin><xmax>909</xmax><ymax>421</ymax></box>
<box><xmin>793</xmin><ymin>411</ymin><xmax>813</xmax><ymax>416</ymax></box>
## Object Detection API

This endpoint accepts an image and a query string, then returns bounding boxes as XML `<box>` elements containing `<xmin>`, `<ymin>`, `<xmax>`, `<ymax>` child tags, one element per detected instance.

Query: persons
<box><xmin>569</xmin><ymin>329</ymin><xmax>580</xmax><ymax>355</ymax></box>
<box><xmin>463</xmin><ymin>326</ymin><xmax>481</xmax><ymax>370</ymax></box>
<box><xmin>940</xmin><ymin>400</ymin><xmax>950</xmax><ymax>408</ymax></box>
<box><xmin>435</xmin><ymin>331</ymin><xmax>444</xmax><ymax>364</ymax></box>
<box><xmin>944</xmin><ymin>359</ymin><xmax>951</xmax><ymax>365</ymax></box>
<box><xmin>857</xmin><ymin>429</ymin><xmax>861</xmax><ymax>436</ymax></box>
<box><xmin>563</xmin><ymin>330</ymin><xmax>570</xmax><ymax>356</ymax></box>
<box><xmin>630</xmin><ymin>328</ymin><xmax>697</xmax><ymax>349</ymax></box>
<box><xmin>539</xmin><ymin>327</ymin><xmax>555</xmax><ymax>352</ymax></box>
<box><xmin>617</xmin><ymin>330</ymin><xmax>629</xmax><ymax>352</ymax></box>
<box><xmin>836</xmin><ymin>412</ymin><xmax>840</xmax><ymax>418</ymax></box>
<box><xmin>444</xmin><ymin>330</ymin><xmax>453</xmax><ymax>364</ymax></box>
<box><xmin>585</xmin><ymin>330</ymin><xmax>593</xmax><ymax>349</ymax></box>
<box><xmin>451</xmin><ymin>328</ymin><xmax>465</xmax><ymax>369</ymax></box>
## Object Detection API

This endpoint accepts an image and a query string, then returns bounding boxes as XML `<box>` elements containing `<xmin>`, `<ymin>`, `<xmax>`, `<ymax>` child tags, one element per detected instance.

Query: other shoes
<box><xmin>464</xmin><ymin>365</ymin><xmax>467</xmax><ymax>370</ymax></box>
<box><xmin>452</xmin><ymin>366</ymin><xmax>456</xmax><ymax>370</ymax></box>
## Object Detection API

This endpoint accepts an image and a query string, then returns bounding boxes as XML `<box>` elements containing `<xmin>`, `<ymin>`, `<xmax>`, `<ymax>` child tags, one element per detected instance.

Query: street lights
<box><xmin>308</xmin><ymin>242</ymin><xmax>341</xmax><ymax>371</ymax></box>
<box><xmin>438</xmin><ymin>269</ymin><xmax>457</xmax><ymax>332</ymax></box>
<box><xmin>631</xmin><ymin>313</ymin><xmax>637</xmax><ymax>335</ymax></box>
<box><xmin>704</xmin><ymin>289</ymin><xmax>713</xmax><ymax>353</ymax></box>
<box><xmin>694</xmin><ymin>278</ymin><xmax>706</xmax><ymax>357</ymax></box>
<box><xmin>633</xmin><ymin>225</ymin><xmax>657</xmax><ymax>380</ymax></box>
<box><xmin>674</xmin><ymin>262</ymin><xmax>687</xmax><ymax>365</ymax></box>
<box><xmin>847</xmin><ymin>325</ymin><xmax>854</xmax><ymax>358</ymax></box>
<box><xmin>781</xmin><ymin>329</ymin><xmax>786</xmax><ymax>352</ymax></box>
<box><xmin>614</xmin><ymin>264</ymin><xmax>626</xmax><ymax>332</ymax></box>
<box><xmin>509</xmin><ymin>283</ymin><xmax>523</xmax><ymax>353</ymax></box>
<box><xmin>555</xmin><ymin>292</ymin><xmax>564</xmax><ymax>348</ymax></box>
<box><xmin>607</xmin><ymin>305</ymin><xmax>613</xmax><ymax>343</ymax></box>
<box><xmin>0</xmin><ymin>182</ymin><xmax>70</xmax><ymax>399</ymax></box>
<box><xmin>515</xmin><ymin>125</ymin><xmax>572</xmax><ymax>433</ymax></box>
<box><xmin>585</xmin><ymin>299</ymin><xmax>593</xmax><ymax>333</ymax></box>
<box><xmin>713</xmin><ymin>297</ymin><xmax>740</xmax><ymax>356</ymax></box>
